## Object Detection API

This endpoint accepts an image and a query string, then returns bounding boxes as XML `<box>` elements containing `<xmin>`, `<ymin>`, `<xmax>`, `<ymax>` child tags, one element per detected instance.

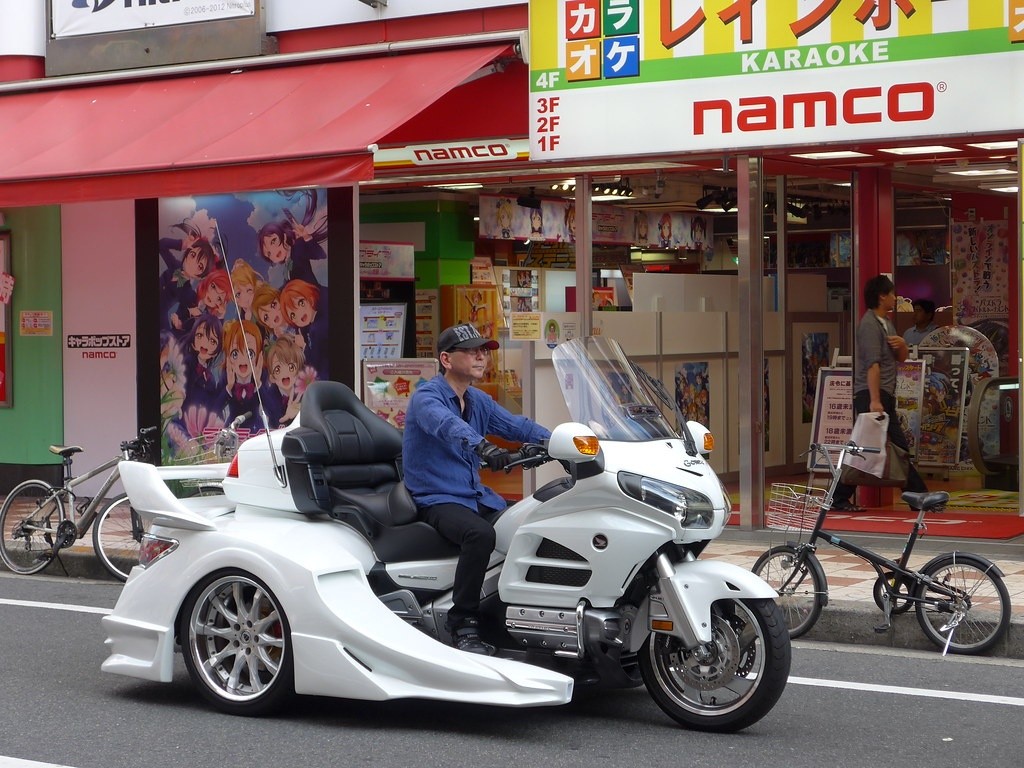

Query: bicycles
<box><xmin>1</xmin><ymin>421</ymin><xmax>155</xmax><ymax>581</ymax></box>
<box><xmin>216</xmin><ymin>411</ymin><xmax>249</xmax><ymax>462</ymax></box>
<box><xmin>753</xmin><ymin>438</ymin><xmax>1009</xmax><ymax>657</ymax></box>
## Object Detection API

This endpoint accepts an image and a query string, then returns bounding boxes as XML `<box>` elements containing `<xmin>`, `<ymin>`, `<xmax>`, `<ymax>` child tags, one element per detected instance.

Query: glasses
<box><xmin>445</xmin><ymin>347</ymin><xmax>490</xmax><ymax>356</ymax></box>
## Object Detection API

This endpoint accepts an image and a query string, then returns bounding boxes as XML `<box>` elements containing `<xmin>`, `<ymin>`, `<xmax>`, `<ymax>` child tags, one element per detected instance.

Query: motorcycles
<box><xmin>98</xmin><ymin>331</ymin><xmax>793</xmax><ymax>731</ymax></box>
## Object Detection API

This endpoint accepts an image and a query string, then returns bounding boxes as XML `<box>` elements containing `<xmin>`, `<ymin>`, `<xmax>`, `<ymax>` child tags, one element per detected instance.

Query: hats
<box><xmin>438</xmin><ymin>323</ymin><xmax>500</xmax><ymax>356</ymax></box>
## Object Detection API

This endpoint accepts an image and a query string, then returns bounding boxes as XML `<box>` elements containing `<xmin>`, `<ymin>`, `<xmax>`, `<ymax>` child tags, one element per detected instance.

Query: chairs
<box><xmin>282</xmin><ymin>381</ymin><xmax>510</xmax><ymax>563</ymax></box>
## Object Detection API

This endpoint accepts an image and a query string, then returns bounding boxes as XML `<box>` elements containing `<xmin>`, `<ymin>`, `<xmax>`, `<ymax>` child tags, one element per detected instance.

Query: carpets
<box><xmin>726</xmin><ymin>501</ymin><xmax>1024</xmax><ymax>540</ymax></box>
<box><xmin>944</xmin><ymin>489</ymin><xmax>1019</xmax><ymax>508</ymax></box>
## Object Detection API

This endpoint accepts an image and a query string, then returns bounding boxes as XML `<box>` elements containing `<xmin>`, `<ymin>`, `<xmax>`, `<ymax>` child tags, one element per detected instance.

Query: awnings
<box><xmin>0</xmin><ymin>44</ymin><xmax>510</xmax><ymax>208</ymax></box>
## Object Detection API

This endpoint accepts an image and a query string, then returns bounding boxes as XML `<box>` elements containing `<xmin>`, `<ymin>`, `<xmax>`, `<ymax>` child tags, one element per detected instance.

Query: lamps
<box><xmin>977</xmin><ymin>182</ymin><xmax>1018</xmax><ymax>193</ymax></box>
<box><xmin>937</xmin><ymin>162</ymin><xmax>1018</xmax><ymax>176</ymax></box>
<box><xmin>550</xmin><ymin>178</ymin><xmax>633</xmax><ymax>197</ymax></box>
<box><xmin>695</xmin><ymin>185</ymin><xmax>823</xmax><ymax>221</ymax></box>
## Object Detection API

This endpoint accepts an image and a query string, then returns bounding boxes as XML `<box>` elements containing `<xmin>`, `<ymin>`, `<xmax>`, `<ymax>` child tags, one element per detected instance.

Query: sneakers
<box><xmin>444</xmin><ymin>618</ymin><xmax>489</xmax><ymax>655</ymax></box>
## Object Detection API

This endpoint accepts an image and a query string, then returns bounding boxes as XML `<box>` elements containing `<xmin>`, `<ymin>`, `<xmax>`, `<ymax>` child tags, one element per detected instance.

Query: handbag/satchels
<box><xmin>842</xmin><ymin>412</ymin><xmax>889</xmax><ymax>478</ymax></box>
<box><xmin>840</xmin><ymin>441</ymin><xmax>914</xmax><ymax>487</ymax></box>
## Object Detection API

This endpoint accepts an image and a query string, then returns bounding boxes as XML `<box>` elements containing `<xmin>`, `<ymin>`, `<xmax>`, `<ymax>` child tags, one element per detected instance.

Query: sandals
<box><xmin>836</xmin><ymin>503</ymin><xmax>867</xmax><ymax>512</ymax></box>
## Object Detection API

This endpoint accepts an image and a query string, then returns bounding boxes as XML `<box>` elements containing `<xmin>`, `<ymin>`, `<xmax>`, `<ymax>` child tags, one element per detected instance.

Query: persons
<box><xmin>826</xmin><ymin>275</ymin><xmax>945</xmax><ymax>512</ymax></box>
<box><xmin>402</xmin><ymin>323</ymin><xmax>552</xmax><ymax>655</ymax></box>
<box><xmin>903</xmin><ymin>299</ymin><xmax>939</xmax><ymax>346</ymax></box>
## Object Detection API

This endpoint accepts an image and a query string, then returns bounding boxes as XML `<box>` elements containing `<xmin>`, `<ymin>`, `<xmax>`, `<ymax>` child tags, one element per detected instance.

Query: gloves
<box><xmin>480</xmin><ymin>443</ymin><xmax>512</xmax><ymax>474</ymax></box>
